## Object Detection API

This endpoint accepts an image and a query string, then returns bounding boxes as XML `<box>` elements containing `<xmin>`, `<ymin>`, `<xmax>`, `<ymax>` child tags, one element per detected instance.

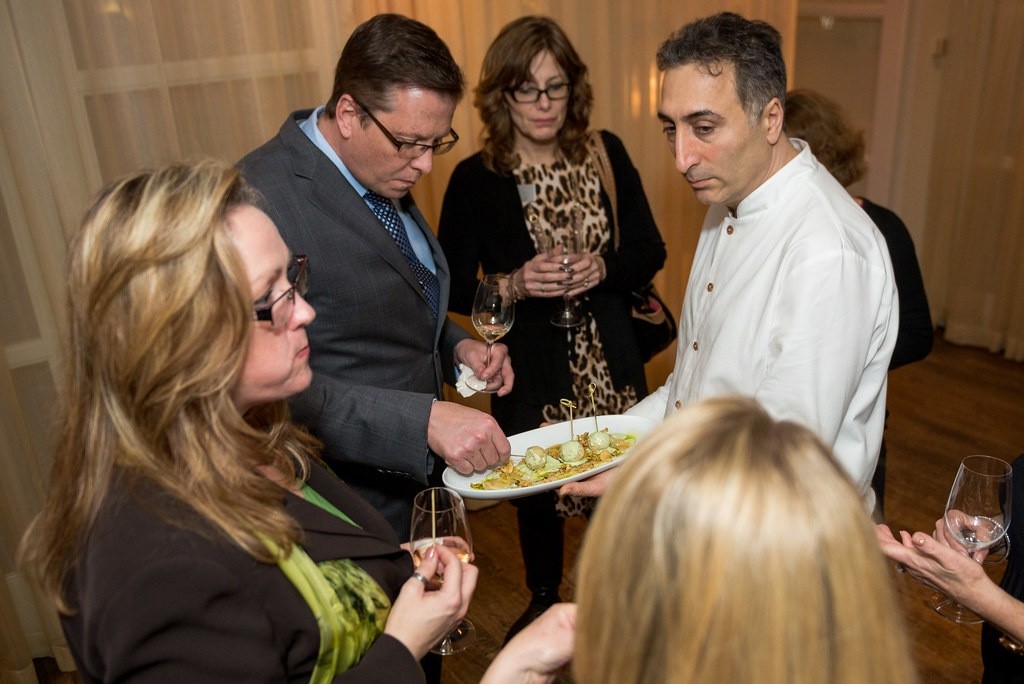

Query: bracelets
<box><xmin>507</xmin><ymin>266</ymin><xmax>525</xmax><ymax>304</ymax></box>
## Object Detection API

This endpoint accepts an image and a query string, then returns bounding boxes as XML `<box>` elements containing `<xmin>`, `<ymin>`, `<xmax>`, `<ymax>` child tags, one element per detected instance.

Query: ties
<box><xmin>364</xmin><ymin>191</ymin><xmax>439</xmax><ymax>321</ymax></box>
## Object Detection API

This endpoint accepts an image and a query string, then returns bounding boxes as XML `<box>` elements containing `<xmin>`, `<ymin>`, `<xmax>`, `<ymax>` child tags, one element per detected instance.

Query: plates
<box><xmin>441</xmin><ymin>414</ymin><xmax>655</xmax><ymax>499</ymax></box>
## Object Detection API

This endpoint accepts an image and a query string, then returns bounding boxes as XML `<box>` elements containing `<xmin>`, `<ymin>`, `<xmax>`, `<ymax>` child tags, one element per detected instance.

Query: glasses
<box><xmin>352</xmin><ymin>96</ymin><xmax>458</xmax><ymax>159</ymax></box>
<box><xmin>251</xmin><ymin>253</ymin><xmax>311</xmax><ymax>327</ymax></box>
<box><xmin>506</xmin><ymin>82</ymin><xmax>571</xmax><ymax>103</ymax></box>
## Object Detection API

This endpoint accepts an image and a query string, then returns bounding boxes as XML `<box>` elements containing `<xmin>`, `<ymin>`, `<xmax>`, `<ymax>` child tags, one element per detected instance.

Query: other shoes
<box><xmin>502</xmin><ymin>593</ymin><xmax>561</xmax><ymax>647</ymax></box>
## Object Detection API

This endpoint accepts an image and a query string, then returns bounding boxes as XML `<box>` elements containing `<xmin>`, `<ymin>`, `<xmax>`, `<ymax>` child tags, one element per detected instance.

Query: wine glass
<box><xmin>928</xmin><ymin>455</ymin><xmax>1013</xmax><ymax>624</ymax></box>
<box><xmin>465</xmin><ymin>275</ymin><xmax>515</xmax><ymax>393</ymax></box>
<box><xmin>550</xmin><ymin>225</ymin><xmax>586</xmax><ymax>327</ymax></box>
<box><xmin>410</xmin><ymin>487</ymin><xmax>475</xmax><ymax>656</ymax></box>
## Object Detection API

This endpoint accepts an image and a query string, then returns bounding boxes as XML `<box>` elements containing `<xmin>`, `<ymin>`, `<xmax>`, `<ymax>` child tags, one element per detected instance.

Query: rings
<box><xmin>895</xmin><ymin>561</ymin><xmax>906</xmax><ymax>574</ymax></box>
<box><xmin>583</xmin><ymin>277</ymin><xmax>589</xmax><ymax>287</ymax></box>
<box><xmin>410</xmin><ymin>572</ymin><xmax>428</xmax><ymax>587</ymax></box>
<box><xmin>541</xmin><ymin>282</ymin><xmax>545</xmax><ymax>292</ymax></box>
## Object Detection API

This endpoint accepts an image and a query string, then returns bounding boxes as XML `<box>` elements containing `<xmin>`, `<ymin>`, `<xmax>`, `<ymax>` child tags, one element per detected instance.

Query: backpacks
<box><xmin>635</xmin><ymin>285</ymin><xmax>676</xmax><ymax>362</ymax></box>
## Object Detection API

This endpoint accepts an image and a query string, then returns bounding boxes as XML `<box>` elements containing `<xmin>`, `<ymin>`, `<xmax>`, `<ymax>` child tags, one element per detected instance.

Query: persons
<box><xmin>225</xmin><ymin>13</ymin><xmax>521</xmax><ymax>684</ymax></box>
<box><xmin>558</xmin><ymin>10</ymin><xmax>904</xmax><ymax>545</ymax></box>
<box><xmin>16</xmin><ymin>158</ymin><xmax>479</xmax><ymax>684</ymax></box>
<box><xmin>434</xmin><ymin>15</ymin><xmax>667</xmax><ymax>651</ymax></box>
<box><xmin>866</xmin><ymin>453</ymin><xmax>1024</xmax><ymax>683</ymax></box>
<box><xmin>479</xmin><ymin>395</ymin><xmax>924</xmax><ymax>683</ymax></box>
<box><xmin>779</xmin><ymin>86</ymin><xmax>936</xmax><ymax>526</ymax></box>
